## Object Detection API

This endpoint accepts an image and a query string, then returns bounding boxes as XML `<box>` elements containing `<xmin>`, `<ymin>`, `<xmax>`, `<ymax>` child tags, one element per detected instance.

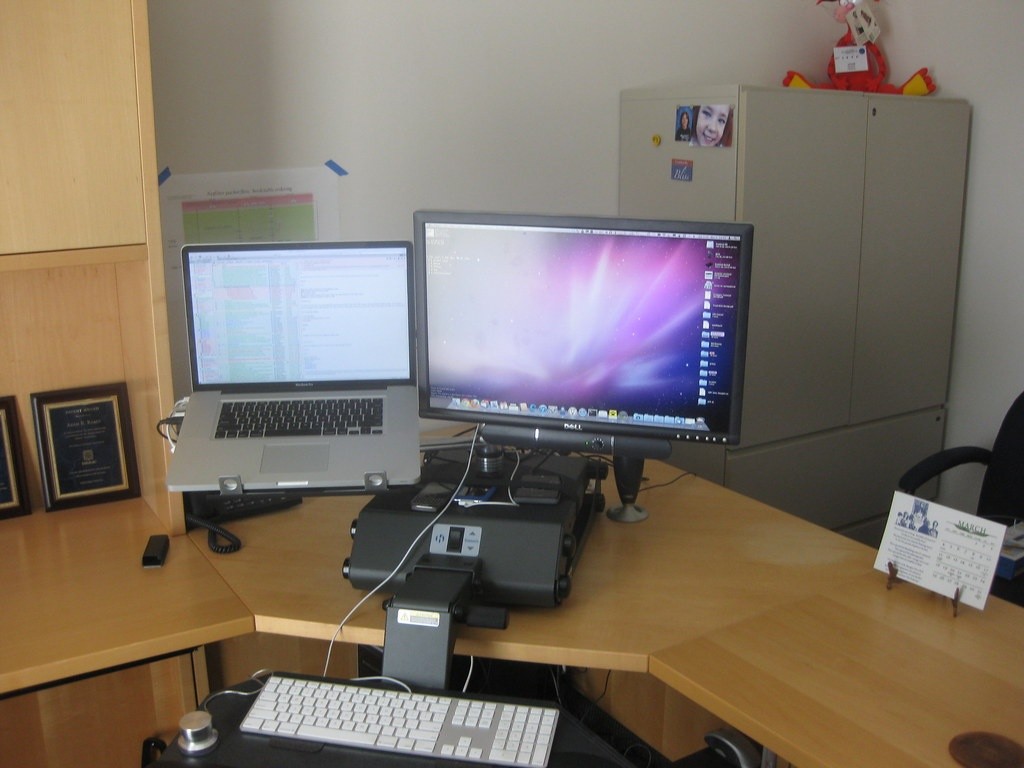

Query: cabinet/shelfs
<box><xmin>649</xmin><ymin>406</ymin><xmax>946</xmax><ymax>550</ymax></box>
<box><xmin>0</xmin><ymin>0</ymin><xmax>188</xmax><ymax>549</ymax></box>
<box><xmin>619</xmin><ymin>82</ymin><xmax>972</xmax><ymax>447</ymax></box>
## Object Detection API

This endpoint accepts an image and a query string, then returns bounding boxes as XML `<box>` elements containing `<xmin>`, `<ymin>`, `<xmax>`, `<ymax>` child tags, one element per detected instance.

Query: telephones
<box><xmin>183</xmin><ymin>492</ymin><xmax>303</xmax><ymax>530</ymax></box>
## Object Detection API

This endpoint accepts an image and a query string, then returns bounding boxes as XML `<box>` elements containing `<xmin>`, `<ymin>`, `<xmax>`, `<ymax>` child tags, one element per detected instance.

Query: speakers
<box><xmin>607</xmin><ymin>459</ymin><xmax>649</xmax><ymax>523</ymax></box>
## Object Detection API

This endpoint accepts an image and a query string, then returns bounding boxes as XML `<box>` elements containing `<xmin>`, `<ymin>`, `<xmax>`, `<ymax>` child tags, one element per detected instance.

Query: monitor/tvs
<box><xmin>414</xmin><ymin>209</ymin><xmax>754</xmax><ymax>459</ymax></box>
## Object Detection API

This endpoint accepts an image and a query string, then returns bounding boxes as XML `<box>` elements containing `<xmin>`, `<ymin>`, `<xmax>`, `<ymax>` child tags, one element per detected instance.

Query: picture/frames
<box><xmin>0</xmin><ymin>395</ymin><xmax>32</xmax><ymax>520</ymax></box>
<box><xmin>30</xmin><ymin>381</ymin><xmax>142</xmax><ymax>513</ymax></box>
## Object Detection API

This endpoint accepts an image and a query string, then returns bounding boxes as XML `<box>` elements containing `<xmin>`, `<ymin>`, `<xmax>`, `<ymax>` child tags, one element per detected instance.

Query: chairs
<box><xmin>898</xmin><ymin>391</ymin><xmax>1024</xmax><ymax>610</ymax></box>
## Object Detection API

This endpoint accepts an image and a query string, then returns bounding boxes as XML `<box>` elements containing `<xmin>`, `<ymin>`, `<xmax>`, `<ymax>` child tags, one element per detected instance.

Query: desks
<box><xmin>648</xmin><ymin>565</ymin><xmax>1024</xmax><ymax>768</ymax></box>
<box><xmin>1</xmin><ymin>489</ymin><xmax>255</xmax><ymax>767</ymax></box>
<box><xmin>190</xmin><ymin>459</ymin><xmax>879</xmax><ymax>767</ymax></box>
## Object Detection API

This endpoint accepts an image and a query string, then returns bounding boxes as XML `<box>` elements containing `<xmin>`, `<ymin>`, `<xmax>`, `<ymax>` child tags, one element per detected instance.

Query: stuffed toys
<box><xmin>783</xmin><ymin>0</ymin><xmax>937</xmax><ymax>97</ymax></box>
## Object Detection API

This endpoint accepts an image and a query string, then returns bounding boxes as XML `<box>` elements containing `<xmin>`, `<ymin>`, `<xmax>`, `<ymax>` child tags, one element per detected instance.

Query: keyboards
<box><xmin>240</xmin><ymin>675</ymin><xmax>559</xmax><ymax>768</ymax></box>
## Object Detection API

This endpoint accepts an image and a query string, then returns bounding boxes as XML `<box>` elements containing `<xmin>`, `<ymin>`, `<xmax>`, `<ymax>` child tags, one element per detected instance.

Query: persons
<box><xmin>676</xmin><ymin>112</ymin><xmax>691</xmax><ymax>141</ymax></box>
<box><xmin>693</xmin><ymin>105</ymin><xmax>732</xmax><ymax>146</ymax></box>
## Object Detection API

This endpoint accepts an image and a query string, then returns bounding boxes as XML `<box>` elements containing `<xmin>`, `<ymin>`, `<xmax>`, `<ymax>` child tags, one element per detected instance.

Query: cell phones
<box><xmin>411</xmin><ymin>482</ymin><xmax>456</xmax><ymax>512</ymax></box>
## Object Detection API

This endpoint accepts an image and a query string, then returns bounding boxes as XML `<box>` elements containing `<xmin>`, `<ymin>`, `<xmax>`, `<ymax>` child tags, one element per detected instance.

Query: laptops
<box><xmin>168</xmin><ymin>240</ymin><xmax>421</xmax><ymax>492</ymax></box>
